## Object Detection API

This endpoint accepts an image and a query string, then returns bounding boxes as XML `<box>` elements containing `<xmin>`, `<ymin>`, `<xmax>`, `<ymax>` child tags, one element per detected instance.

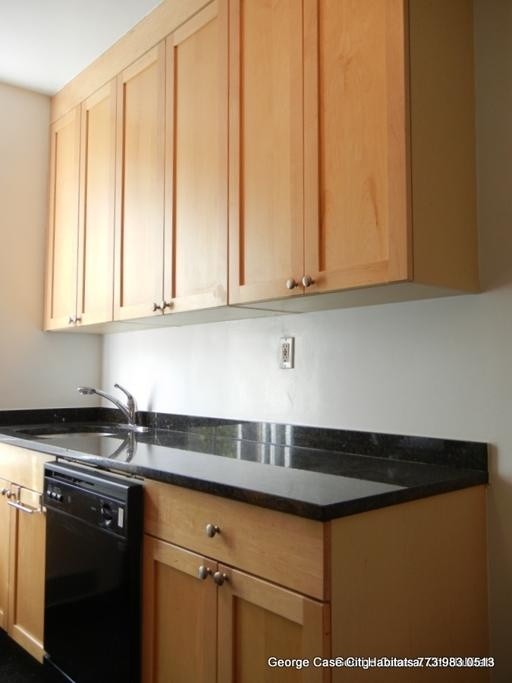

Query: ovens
<box><xmin>41</xmin><ymin>458</ymin><xmax>143</xmax><ymax>682</ymax></box>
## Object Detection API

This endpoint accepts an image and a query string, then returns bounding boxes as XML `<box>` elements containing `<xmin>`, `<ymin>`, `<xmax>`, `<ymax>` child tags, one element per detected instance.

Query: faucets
<box><xmin>76</xmin><ymin>384</ymin><xmax>135</xmax><ymax>424</ymax></box>
<box><xmin>108</xmin><ymin>431</ymin><xmax>135</xmax><ymax>462</ymax></box>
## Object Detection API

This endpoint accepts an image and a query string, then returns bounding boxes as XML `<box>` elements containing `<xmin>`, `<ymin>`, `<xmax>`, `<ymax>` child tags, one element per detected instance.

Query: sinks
<box><xmin>15</xmin><ymin>426</ymin><xmax>118</xmax><ymax>439</ymax></box>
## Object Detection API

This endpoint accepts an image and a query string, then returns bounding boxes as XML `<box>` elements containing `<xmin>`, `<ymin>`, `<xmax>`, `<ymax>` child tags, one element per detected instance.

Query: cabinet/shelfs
<box><xmin>0</xmin><ymin>443</ymin><xmax>58</xmax><ymax>664</ymax></box>
<box><xmin>43</xmin><ymin>0</ymin><xmax>479</xmax><ymax>336</ymax></box>
<box><xmin>144</xmin><ymin>478</ymin><xmax>489</xmax><ymax>683</ymax></box>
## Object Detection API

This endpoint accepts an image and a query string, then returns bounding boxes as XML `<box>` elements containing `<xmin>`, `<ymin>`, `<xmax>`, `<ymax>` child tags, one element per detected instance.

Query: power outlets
<box><xmin>279</xmin><ymin>337</ymin><xmax>294</xmax><ymax>369</ymax></box>
